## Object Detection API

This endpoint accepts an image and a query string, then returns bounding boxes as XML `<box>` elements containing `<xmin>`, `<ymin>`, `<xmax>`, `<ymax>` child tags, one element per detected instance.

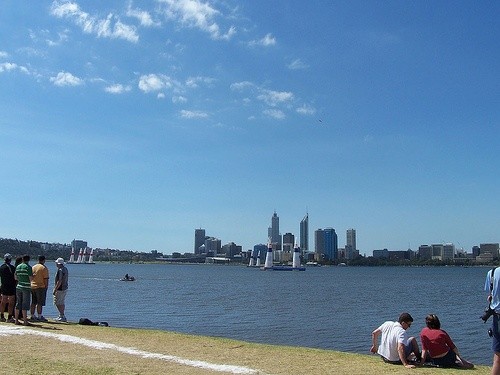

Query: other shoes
<box><xmin>22</xmin><ymin>318</ymin><xmax>29</xmax><ymax>321</ymax></box>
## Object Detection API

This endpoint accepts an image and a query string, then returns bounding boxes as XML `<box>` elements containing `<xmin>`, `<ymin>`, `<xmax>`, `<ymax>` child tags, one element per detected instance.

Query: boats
<box><xmin>119</xmin><ymin>273</ymin><xmax>135</xmax><ymax>281</ymax></box>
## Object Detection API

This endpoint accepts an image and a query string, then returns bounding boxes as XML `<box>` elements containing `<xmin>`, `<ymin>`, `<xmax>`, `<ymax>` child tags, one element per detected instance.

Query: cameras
<box><xmin>480</xmin><ymin>305</ymin><xmax>494</xmax><ymax>323</ymax></box>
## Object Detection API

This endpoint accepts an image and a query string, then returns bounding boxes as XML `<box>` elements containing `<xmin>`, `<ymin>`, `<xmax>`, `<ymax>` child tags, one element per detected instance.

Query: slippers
<box><xmin>23</xmin><ymin>322</ymin><xmax>34</xmax><ymax>325</ymax></box>
<box><xmin>15</xmin><ymin>322</ymin><xmax>23</xmax><ymax>325</ymax></box>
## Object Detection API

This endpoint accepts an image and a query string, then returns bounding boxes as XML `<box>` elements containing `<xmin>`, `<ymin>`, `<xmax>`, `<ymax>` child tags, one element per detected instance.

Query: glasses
<box><xmin>405</xmin><ymin>321</ymin><xmax>412</xmax><ymax>327</ymax></box>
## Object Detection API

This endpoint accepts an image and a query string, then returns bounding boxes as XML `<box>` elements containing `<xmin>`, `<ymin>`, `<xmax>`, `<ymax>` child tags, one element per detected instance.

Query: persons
<box><xmin>418</xmin><ymin>314</ymin><xmax>475</xmax><ymax>371</ymax></box>
<box><xmin>369</xmin><ymin>312</ymin><xmax>423</xmax><ymax>369</ymax></box>
<box><xmin>0</xmin><ymin>253</ymin><xmax>49</xmax><ymax>325</ymax></box>
<box><xmin>52</xmin><ymin>257</ymin><xmax>69</xmax><ymax>322</ymax></box>
<box><xmin>484</xmin><ymin>263</ymin><xmax>500</xmax><ymax>374</ymax></box>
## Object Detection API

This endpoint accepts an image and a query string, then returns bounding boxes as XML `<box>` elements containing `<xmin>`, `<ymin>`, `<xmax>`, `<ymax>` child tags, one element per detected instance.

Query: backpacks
<box><xmin>79</xmin><ymin>318</ymin><xmax>94</xmax><ymax>325</ymax></box>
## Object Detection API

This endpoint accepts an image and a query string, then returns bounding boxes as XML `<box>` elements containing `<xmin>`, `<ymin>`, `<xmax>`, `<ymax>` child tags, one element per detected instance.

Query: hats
<box><xmin>55</xmin><ymin>258</ymin><xmax>66</xmax><ymax>265</ymax></box>
<box><xmin>23</xmin><ymin>254</ymin><xmax>30</xmax><ymax>261</ymax></box>
<box><xmin>4</xmin><ymin>253</ymin><xmax>12</xmax><ymax>259</ymax></box>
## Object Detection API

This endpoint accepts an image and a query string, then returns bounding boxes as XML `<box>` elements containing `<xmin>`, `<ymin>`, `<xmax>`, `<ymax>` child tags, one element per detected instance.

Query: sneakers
<box><xmin>7</xmin><ymin>318</ymin><xmax>16</xmax><ymax>322</ymax></box>
<box><xmin>53</xmin><ymin>316</ymin><xmax>67</xmax><ymax>322</ymax></box>
<box><xmin>0</xmin><ymin>317</ymin><xmax>6</xmax><ymax>321</ymax></box>
<box><xmin>30</xmin><ymin>316</ymin><xmax>49</xmax><ymax>322</ymax></box>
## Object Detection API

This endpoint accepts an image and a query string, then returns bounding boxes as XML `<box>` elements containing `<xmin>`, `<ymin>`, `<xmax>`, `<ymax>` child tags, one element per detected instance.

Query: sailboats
<box><xmin>66</xmin><ymin>247</ymin><xmax>95</xmax><ymax>264</ymax></box>
<box><xmin>247</xmin><ymin>239</ymin><xmax>307</xmax><ymax>271</ymax></box>
